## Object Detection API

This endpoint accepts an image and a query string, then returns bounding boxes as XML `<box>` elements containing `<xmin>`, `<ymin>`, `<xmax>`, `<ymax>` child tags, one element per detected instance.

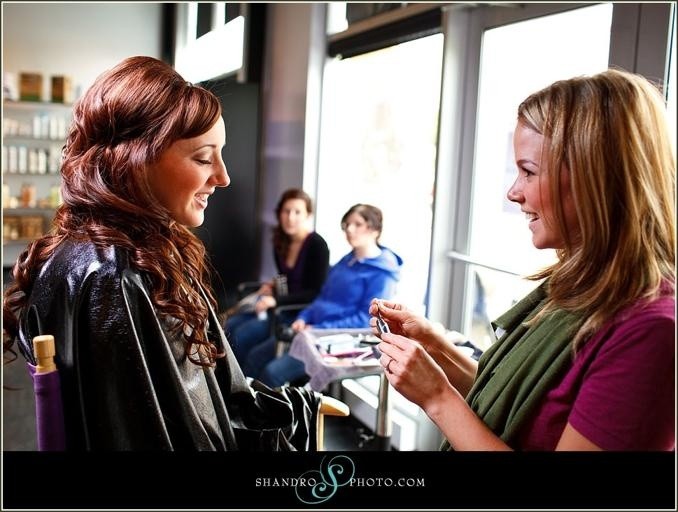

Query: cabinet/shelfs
<box><xmin>1</xmin><ymin>100</ymin><xmax>76</xmax><ymax>271</ymax></box>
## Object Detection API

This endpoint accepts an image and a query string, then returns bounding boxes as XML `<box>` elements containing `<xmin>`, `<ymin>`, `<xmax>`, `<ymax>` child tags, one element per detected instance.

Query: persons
<box><xmin>242</xmin><ymin>204</ymin><xmax>405</xmax><ymax>388</ymax></box>
<box><xmin>369</xmin><ymin>71</ymin><xmax>675</xmax><ymax>451</ymax></box>
<box><xmin>4</xmin><ymin>56</ymin><xmax>322</xmax><ymax>452</ymax></box>
<box><xmin>221</xmin><ymin>190</ymin><xmax>328</xmax><ymax>363</ymax></box>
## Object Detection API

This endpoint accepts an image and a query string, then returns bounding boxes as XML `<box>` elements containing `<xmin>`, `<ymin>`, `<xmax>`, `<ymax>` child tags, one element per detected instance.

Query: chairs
<box><xmin>238</xmin><ymin>264</ymin><xmax>393</xmax><ymax>453</ymax></box>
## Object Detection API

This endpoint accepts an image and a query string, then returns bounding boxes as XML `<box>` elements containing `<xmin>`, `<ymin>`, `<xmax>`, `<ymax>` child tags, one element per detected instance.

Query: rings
<box><xmin>384</xmin><ymin>358</ymin><xmax>395</xmax><ymax>373</ymax></box>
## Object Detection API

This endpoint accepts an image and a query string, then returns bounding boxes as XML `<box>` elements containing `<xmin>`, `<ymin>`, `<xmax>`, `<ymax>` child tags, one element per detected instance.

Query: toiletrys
<box><xmin>4</xmin><ymin>111</ymin><xmax>65</xmax><ymax>211</ymax></box>
